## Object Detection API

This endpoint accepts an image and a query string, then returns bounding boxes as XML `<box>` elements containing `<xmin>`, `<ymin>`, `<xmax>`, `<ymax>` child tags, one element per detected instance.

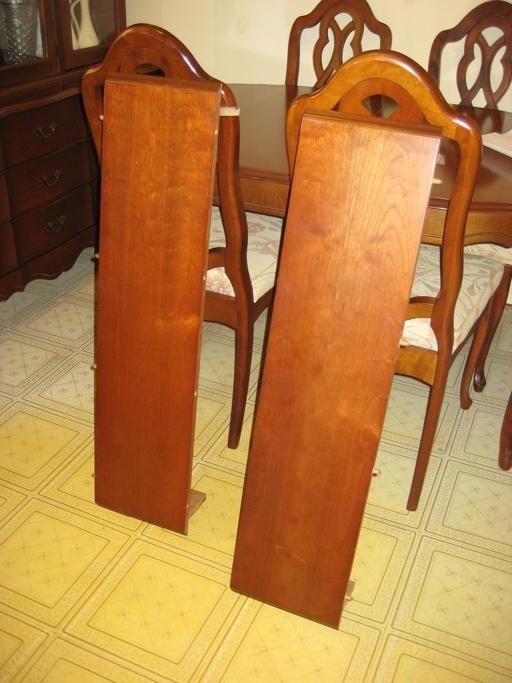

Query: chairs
<box><xmin>288</xmin><ymin>0</ymin><xmax>392</xmax><ymax>85</ymax></box>
<box><xmin>430</xmin><ymin>0</ymin><xmax>512</xmax><ymax>392</ymax></box>
<box><xmin>86</xmin><ymin>24</ymin><xmax>284</xmax><ymax>447</ymax></box>
<box><xmin>283</xmin><ymin>50</ymin><xmax>503</xmax><ymax>510</ymax></box>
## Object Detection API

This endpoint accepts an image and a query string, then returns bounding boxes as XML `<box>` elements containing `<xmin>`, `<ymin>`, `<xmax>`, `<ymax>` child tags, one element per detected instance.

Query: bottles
<box><xmin>70</xmin><ymin>0</ymin><xmax>100</xmax><ymax>50</ymax></box>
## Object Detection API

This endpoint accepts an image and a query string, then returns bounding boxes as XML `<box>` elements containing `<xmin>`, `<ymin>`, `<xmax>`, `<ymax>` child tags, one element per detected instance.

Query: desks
<box><xmin>216</xmin><ymin>85</ymin><xmax>511</xmax><ymax>474</ymax></box>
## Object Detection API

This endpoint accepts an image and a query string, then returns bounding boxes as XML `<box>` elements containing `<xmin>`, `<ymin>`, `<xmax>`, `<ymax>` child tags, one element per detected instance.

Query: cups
<box><xmin>1</xmin><ymin>0</ymin><xmax>39</xmax><ymax>67</ymax></box>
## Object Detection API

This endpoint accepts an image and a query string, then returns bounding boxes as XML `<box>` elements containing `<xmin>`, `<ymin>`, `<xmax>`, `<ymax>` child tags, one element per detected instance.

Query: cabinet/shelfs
<box><xmin>0</xmin><ymin>0</ymin><xmax>126</xmax><ymax>301</ymax></box>
